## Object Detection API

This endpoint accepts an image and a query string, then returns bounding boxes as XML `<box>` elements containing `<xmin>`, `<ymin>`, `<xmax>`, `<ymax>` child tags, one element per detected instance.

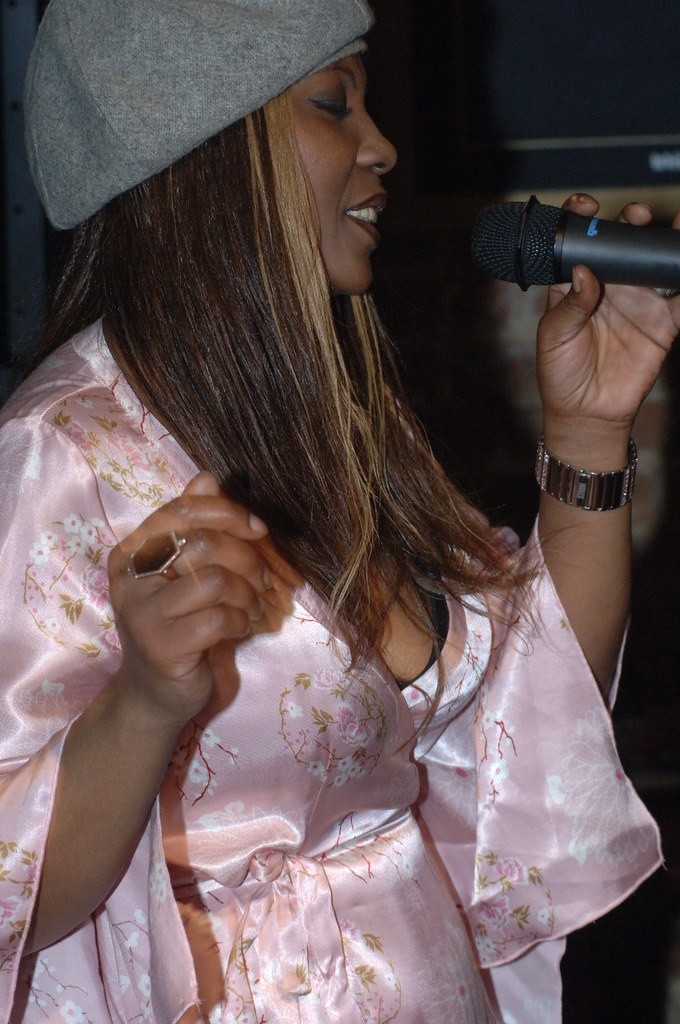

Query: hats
<box><xmin>24</xmin><ymin>0</ymin><xmax>375</xmax><ymax>230</ymax></box>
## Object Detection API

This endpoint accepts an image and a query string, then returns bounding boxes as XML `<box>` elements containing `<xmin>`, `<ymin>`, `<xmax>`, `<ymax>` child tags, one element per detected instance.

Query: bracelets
<box><xmin>535</xmin><ymin>429</ymin><xmax>638</xmax><ymax>511</ymax></box>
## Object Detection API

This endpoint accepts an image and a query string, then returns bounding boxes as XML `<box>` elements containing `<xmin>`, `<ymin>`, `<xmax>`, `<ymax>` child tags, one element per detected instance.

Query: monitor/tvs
<box><xmin>408</xmin><ymin>0</ymin><xmax>680</xmax><ymax>193</ymax></box>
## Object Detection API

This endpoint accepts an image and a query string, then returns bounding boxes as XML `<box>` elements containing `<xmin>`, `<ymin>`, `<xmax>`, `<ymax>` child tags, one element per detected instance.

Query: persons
<box><xmin>0</xmin><ymin>2</ymin><xmax>680</xmax><ymax>1024</ymax></box>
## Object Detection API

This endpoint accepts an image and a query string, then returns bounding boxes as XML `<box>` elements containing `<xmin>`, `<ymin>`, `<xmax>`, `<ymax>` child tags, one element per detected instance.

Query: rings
<box><xmin>654</xmin><ymin>287</ymin><xmax>678</xmax><ymax>298</ymax></box>
<box><xmin>125</xmin><ymin>530</ymin><xmax>189</xmax><ymax>581</ymax></box>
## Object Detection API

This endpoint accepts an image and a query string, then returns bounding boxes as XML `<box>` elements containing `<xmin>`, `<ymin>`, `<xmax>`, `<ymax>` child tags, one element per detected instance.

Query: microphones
<box><xmin>467</xmin><ymin>195</ymin><xmax>679</xmax><ymax>296</ymax></box>
